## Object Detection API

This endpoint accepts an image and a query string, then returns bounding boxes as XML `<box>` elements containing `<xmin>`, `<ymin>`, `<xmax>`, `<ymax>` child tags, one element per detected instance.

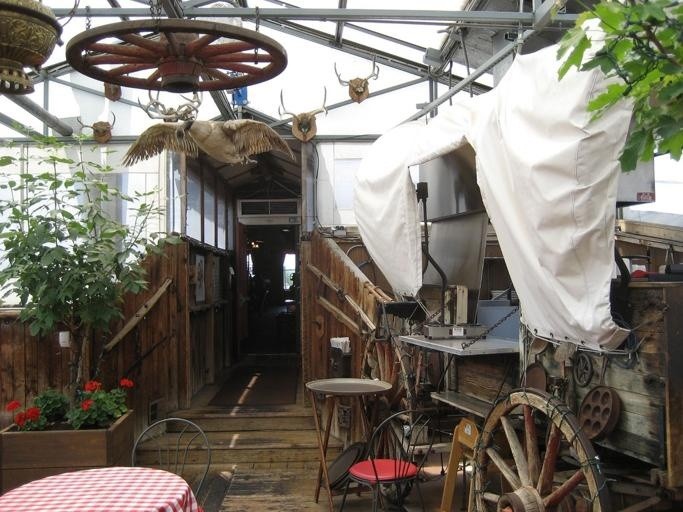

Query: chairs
<box><xmin>337</xmin><ymin>408</ymin><xmax>434</xmax><ymax>511</ymax></box>
<box><xmin>132</xmin><ymin>417</ymin><xmax>210</xmax><ymax>497</ymax></box>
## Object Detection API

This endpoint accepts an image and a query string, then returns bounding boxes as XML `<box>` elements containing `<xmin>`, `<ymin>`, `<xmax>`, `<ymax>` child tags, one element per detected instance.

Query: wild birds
<box><xmin>120</xmin><ymin>116</ymin><xmax>297</xmax><ymax>167</ymax></box>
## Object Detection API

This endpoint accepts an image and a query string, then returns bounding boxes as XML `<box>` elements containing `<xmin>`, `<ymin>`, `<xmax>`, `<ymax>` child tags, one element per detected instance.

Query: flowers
<box><xmin>3</xmin><ymin>378</ymin><xmax>136</xmax><ymax>429</ymax></box>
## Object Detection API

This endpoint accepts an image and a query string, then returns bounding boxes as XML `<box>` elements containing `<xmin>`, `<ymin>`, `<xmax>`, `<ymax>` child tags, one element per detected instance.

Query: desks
<box><xmin>305</xmin><ymin>378</ymin><xmax>392</xmax><ymax>511</ymax></box>
<box><xmin>1</xmin><ymin>466</ymin><xmax>195</xmax><ymax>510</ymax></box>
